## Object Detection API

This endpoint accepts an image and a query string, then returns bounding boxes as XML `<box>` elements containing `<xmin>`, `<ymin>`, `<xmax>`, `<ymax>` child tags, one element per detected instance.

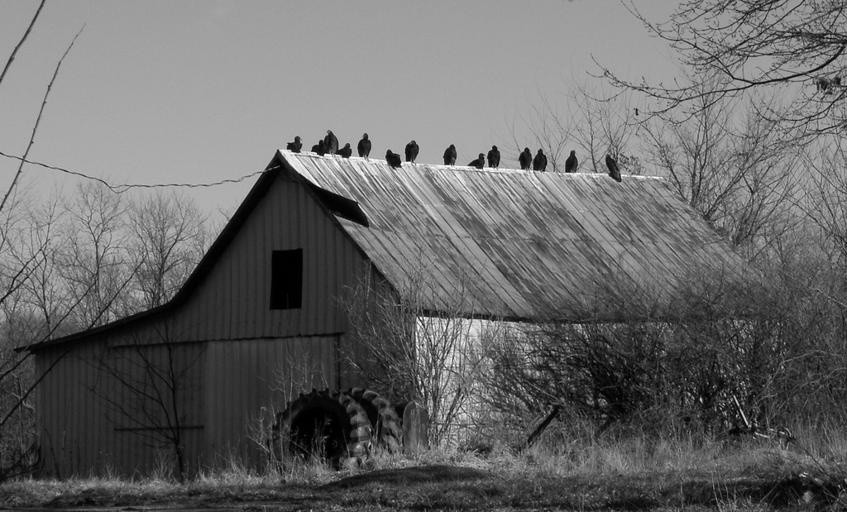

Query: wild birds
<box><xmin>311</xmin><ymin>129</ymin><xmax>352</xmax><ymax>157</ymax></box>
<box><xmin>357</xmin><ymin>133</ymin><xmax>372</xmax><ymax>161</ymax></box>
<box><xmin>565</xmin><ymin>150</ymin><xmax>578</xmax><ymax>172</ymax></box>
<box><xmin>404</xmin><ymin>140</ymin><xmax>420</xmax><ymax>165</ymax></box>
<box><xmin>385</xmin><ymin>149</ymin><xmax>402</xmax><ymax>169</ymax></box>
<box><xmin>286</xmin><ymin>136</ymin><xmax>303</xmax><ymax>152</ymax></box>
<box><xmin>468</xmin><ymin>146</ymin><xmax>501</xmax><ymax>169</ymax></box>
<box><xmin>443</xmin><ymin>144</ymin><xmax>457</xmax><ymax>166</ymax></box>
<box><xmin>605</xmin><ymin>154</ymin><xmax>622</xmax><ymax>183</ymax></box>
<box><xmin>518</xmin><ymin>147</ymin><xmax>547</xmax><ymax>171</ymax></box>
